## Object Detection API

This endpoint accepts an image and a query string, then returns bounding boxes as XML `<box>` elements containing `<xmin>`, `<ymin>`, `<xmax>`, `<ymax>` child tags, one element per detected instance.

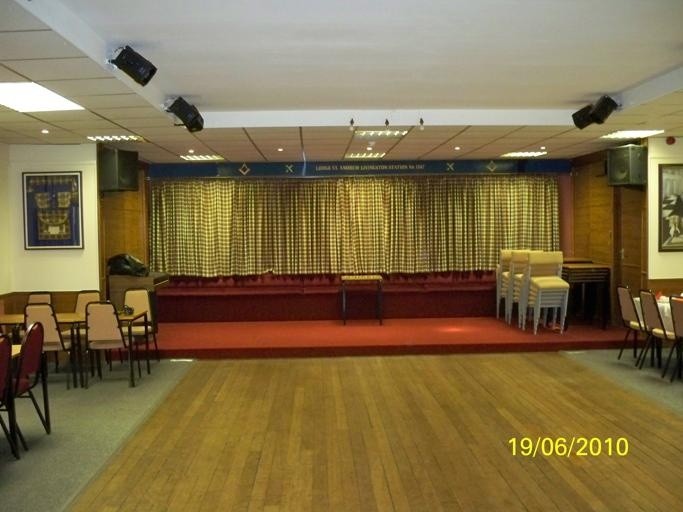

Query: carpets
<box><xmin>556</xmin><ymin>347</ymin><xmax>682</xmax><ymax>421</ymax></box>
<box><xmin>0</xmin><ymin>357</ymin><xmax>197</xmax><ymax>512</ymax></box>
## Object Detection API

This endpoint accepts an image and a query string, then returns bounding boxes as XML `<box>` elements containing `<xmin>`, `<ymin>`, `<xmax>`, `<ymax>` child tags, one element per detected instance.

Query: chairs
<box><xmin>0</xmin><ymin>288</ymin><xmax>159</xmax><ymax>461</ymax></box>
<box><xmin>494</xmin><ymin>249</ymin><xmax>682</xmax><ymax>385</ymax></box>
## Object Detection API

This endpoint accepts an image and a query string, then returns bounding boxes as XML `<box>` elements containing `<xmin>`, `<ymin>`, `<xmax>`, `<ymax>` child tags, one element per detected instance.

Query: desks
<box><xmin>340</xmin><ymin>274</ymin><xmax>384</xmax><ymax>326</ymax></box>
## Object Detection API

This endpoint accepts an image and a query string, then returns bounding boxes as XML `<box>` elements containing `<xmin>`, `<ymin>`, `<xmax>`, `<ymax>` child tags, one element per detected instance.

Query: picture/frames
<box><xmin>657</xmin><ymin>162</ymin><xmax>682</xmax><ymax>253</ymax></box>
<box><xmin>23</xmin><ymin>170</ymin><xmax>83</xmax><ymax>251</ymax></box>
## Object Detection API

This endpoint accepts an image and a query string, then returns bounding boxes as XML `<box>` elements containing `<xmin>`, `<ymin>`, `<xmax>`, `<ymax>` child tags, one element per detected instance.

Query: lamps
<box><xmin>165</xmin><ymin>99</ymin><xmax>220</xmax><ymax>136</ymax></box>
<box><xmin>570</xmin><ymin>93</ymin><xmax>618</xmax><ymax>134</ymax></box>
<box><xmin>102</xmin><ymin>39</ymin><xmax>161</xmax><ymax>92</ymax></box>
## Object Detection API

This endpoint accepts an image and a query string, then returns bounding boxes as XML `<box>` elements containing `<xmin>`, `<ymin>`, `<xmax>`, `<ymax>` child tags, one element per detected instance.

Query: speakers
<box><xmin>100</xmin><ymin>148</ymin><xmax>138</xmax><ymax>192</ymax></box>
<box><xmin>607</xmin><ymin>144</ymin><xmax>647</xmax><ymax>187</ymax></box>
